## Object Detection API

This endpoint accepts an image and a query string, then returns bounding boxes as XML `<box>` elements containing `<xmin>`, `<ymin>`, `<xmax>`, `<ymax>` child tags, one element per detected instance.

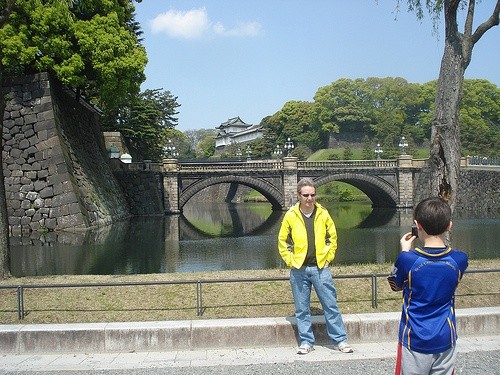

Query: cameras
<box><xmin>412</xmin><ymin>226</ymin><xmax>418</xmax><ymax>238</ymax></box>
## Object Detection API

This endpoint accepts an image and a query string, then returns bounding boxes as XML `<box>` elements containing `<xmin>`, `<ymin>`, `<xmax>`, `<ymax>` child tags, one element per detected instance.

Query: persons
<box><xmin>387</xmin><ymin>197</ymin><xmax>468</xmax><ymax>375</ymax></box>
<box><xmin>278</xmin><ymin>178</ymin><xmax>354</xmax><ymax>353</ymax></box>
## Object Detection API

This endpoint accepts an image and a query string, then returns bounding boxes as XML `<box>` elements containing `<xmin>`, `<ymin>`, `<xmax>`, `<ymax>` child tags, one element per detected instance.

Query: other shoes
<box><xmin>297</xmin><ymin>343</ymin><xmax>314</xmax><ymax>354</ymax></box>
<box><xmin>334</xmin><ymin>341</ymin><xmax>353</xmax><ymax>353</ymax></box>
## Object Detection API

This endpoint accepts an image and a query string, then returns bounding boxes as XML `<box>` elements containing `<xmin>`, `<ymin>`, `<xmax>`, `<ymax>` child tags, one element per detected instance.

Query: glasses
<box><xmin>298</xmin><ymin>192</ymin><xmax>316</xmax><ymax>197</ymax></box>
